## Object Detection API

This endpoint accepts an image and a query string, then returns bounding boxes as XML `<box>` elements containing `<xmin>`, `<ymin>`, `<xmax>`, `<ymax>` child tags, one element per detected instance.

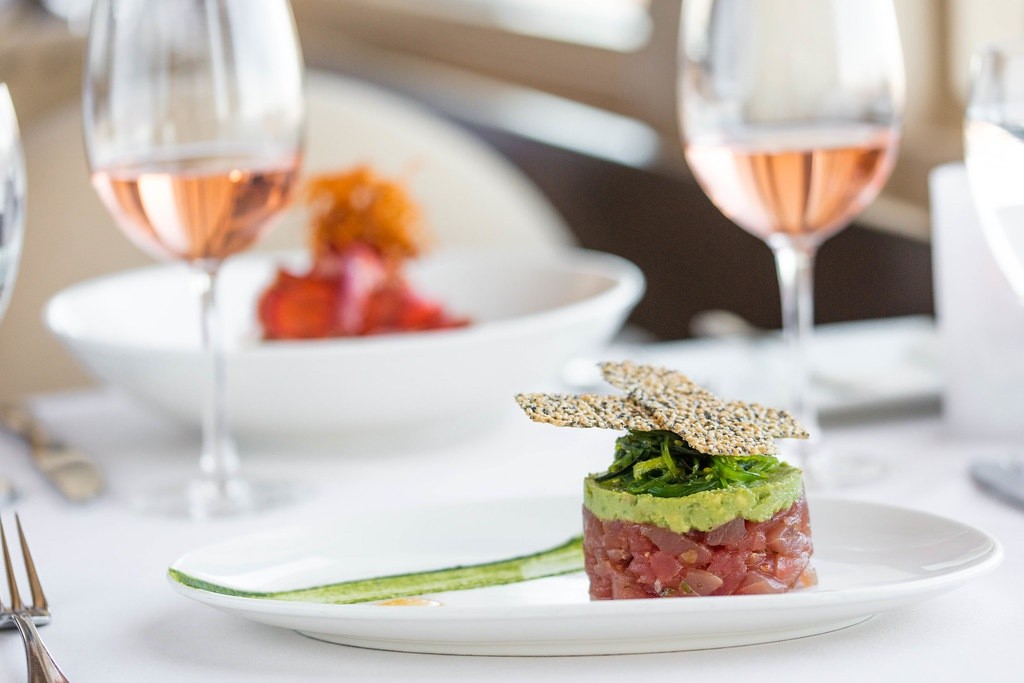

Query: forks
<box><xmin>0</xmin><ymin>510</ymin><xmax>69</xmax><ymax>683</ymax></box>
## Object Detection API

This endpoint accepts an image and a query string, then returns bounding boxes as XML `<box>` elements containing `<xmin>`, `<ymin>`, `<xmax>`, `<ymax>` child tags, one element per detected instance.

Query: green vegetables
<box><xmin>590</xmin><ymin>427</ymin><xmax>777</xmax><ymax>492</ymax></box>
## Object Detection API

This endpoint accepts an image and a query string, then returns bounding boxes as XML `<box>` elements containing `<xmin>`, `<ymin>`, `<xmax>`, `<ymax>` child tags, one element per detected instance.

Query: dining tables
<box><xmin>0</xmin><ymin>315</ymin><xmax>1023</xmax><ymax>683</ymax></box>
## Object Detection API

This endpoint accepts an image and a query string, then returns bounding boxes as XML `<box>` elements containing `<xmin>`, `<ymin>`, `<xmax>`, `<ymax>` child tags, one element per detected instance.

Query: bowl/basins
<box><xmin>50</xmin><ymin>247</ymin><xmax>649</xmax><ymax>446</ymax></box>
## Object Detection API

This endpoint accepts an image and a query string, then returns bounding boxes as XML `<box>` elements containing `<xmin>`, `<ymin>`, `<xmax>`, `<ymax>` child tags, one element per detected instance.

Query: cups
<box><xmin>0</xmin><ymin>81</ymin><xmax>26</xmax><ymax>322</ymax></box>
<box><xmin>926</xmin><ymin>161</ymin><xmax>1024</xmax><ymax>499</ymax></box>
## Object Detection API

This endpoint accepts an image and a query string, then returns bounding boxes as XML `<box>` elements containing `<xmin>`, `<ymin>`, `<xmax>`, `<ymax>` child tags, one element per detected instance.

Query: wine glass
<box><xmin>82</xmin><ymin>0</ymin><xmax>336</xmax><ymax>523</ymax></box>
<box><xmin>674</xmin><ymin>0</ymin><xmax>905</xmax><ymax>478</ymax></box>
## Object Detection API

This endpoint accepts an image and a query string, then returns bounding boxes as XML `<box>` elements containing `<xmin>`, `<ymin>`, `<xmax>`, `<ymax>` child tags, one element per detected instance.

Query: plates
<box><xmin>162</xmin><ymin>496</ymin><xmax>996</xmax><ymax>656</ymax></box>
